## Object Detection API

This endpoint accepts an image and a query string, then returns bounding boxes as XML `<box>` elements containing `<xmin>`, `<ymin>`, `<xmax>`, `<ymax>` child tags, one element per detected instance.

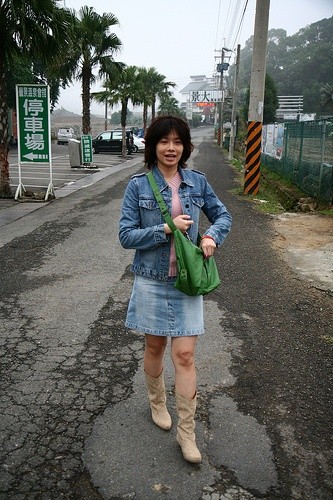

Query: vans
<box><xmin>91</xmin><ymin>130</ymin><xmax>134</xmax><ymax>154</ymax></box>
<box><xmin>57</xmin><ymin>127</ymin><xmax>77</xmax><ymax>145</ymax></box>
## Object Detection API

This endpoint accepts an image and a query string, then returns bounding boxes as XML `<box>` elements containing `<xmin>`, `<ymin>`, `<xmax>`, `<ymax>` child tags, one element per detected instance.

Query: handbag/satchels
<box><xmin>171</xmin><ymin>230</ymin><xmax>220</xmax><ymax>295</ymax></box>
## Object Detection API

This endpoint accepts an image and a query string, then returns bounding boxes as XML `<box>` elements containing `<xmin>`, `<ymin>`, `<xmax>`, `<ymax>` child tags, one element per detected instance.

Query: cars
<box><xmin>132</xmin><ymin>134</ymin><xmax>147</xmax><ymax>153</ymax></box>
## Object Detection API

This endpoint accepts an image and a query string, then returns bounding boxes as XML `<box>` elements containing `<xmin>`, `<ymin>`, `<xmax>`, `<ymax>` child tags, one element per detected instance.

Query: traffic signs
<box><xmin>16</xmin><ymin>84</ymin><xmax>50</xmax><ymax>164</ymax></box>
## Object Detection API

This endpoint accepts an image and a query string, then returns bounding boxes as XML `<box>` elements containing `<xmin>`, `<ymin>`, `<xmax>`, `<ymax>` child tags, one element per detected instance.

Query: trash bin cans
<box><xmin>68</xmin><ymin>138</ymin><xmax>82</xmax><ymax>169</ymax></box>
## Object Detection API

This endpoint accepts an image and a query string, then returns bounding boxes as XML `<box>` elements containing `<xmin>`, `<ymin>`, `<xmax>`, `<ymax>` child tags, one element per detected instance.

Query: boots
<box><xmin>174</xmin><ymin>389</ymin><xmax>202</xmax><ymax>463</ymax></box>
<box><xmin>143</xmin><ymin>368</ymin><xmax>172</xmax><ymax>430</ymax></box>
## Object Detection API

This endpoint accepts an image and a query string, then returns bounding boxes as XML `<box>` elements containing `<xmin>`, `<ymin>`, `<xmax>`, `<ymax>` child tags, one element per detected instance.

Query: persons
<box><xmin>115</xmin><ymin>113</ymin><xmax>234</xmax><ymax>465</ymax></box>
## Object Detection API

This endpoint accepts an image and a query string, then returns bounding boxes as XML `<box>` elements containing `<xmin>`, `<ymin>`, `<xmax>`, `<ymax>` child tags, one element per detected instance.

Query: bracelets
<box><xmin>199</xmin><ymin>236</ymin><xmax>216</xmax><ymax>242</ymax></box>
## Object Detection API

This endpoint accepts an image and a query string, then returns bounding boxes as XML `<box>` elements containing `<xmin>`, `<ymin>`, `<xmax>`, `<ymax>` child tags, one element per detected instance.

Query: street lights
<box><xmin>221</xmin><ymin>46</ymin><xmax>234</xmax><ymax>160</ymax></box>
